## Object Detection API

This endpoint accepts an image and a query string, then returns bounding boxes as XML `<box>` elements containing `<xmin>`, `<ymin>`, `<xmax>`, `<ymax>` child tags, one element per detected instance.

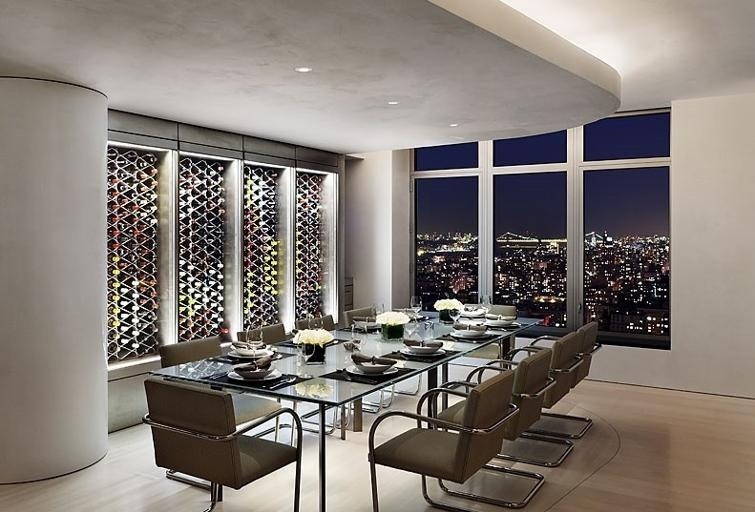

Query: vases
<box><xmin>381</xmin><ymin>324</ymin><xmax>404</xmax><ymax>341</ymax></box>
<box><xmin>302</xmin><ymin>343</ymin><xmax>326</xmax><ymax>364</ymax></box>
<box><xmin>439</xmin><ymin>309</ymin><xmax>458</xmax><ymax>323</ymax></box>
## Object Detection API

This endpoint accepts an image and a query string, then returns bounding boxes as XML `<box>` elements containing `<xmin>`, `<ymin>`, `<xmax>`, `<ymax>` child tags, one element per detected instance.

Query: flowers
<box><xmin>433</xmin><ymin>297</ymin><xmax>466</xmax><ymax>313</ymax></box>
<box><xmin>292</xmin><ymin>328</ymin><xmax>336</xmax><ymax>348</ymax></box>
<box><xmin>375</xmin><ymin>310</ymin><xmax>411</xmax><ymax>326</ymax></box>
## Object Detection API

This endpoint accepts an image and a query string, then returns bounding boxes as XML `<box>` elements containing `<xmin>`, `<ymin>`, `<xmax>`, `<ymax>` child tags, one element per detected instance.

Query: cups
<box><xmin>424</xmin><ymin>321</ymin><xmax>435</xmax><ymax>340</ymax></box>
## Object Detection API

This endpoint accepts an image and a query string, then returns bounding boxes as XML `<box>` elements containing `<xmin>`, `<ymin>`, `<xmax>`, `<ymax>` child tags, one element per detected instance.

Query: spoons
<box><xmin>269</xmin><ymin>376</ymin><xmax>296</xmax><ymax>390</ymax></box>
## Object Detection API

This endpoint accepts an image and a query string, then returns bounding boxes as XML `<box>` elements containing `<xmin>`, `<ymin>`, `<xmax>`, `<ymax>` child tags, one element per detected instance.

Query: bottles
<box><xmin>297</xmin><ymin>315</ymin><xmax>307</xmax><ymax>320</ymax></box>
<box><xmin>108</xmin><ymin>149</ymin><xmax>160</xmax><ymax>361</ymax></box>
<box><xmin>244</xmin><ymin>167</ymin><xmax>279</xmax><ymax>328</ymax></box>
<box><xmin>179</xmin><ymin>156</ymin><xmax>229</xmax><ymax>338</ymax></box>
<box><xmin>295</xmin><ymin>172</ymin><xmax>323</xmax><ymax>314</ymax></box>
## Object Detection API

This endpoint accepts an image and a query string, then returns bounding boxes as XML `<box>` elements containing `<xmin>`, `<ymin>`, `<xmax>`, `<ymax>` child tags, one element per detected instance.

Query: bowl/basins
<box><xmin>487</xmin><ymin>319</ymin><xmax>512</xmax><ymax>325</ymax></box>
<box><xmin>236</xmin><ymin>348</ymin><xmax>265</xmax><ymax>355</ymax></box>
<box><xmin>235</xmin><ymin>368</ymin><xmax>274</xmax><ymax>379</ymax></box>
<box><xmin>356</xmin><ymin>362</ymin><xmax>392</xmax><ymax>373</ymax></box>
<box><xmin>457</xmin><ymin>329</ymin><xmax>485</xmax><ymax>336</ymax></box>
<box><xmin>410</xmin><ymin>346</ymin><xmax>438</xmax><ymax>354</ymax></box>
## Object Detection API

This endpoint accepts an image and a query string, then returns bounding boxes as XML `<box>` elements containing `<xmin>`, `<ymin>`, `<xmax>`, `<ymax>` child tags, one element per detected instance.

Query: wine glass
<box><xmin>308</xmin><ymin>315</ymin><xmax>324</xmax><ymax>330</ymax></box>
<box><xmin>350</xmin><ymin>324</ymin><xmax>368</xmax><ymax>353</ymax></box>
<box><xmin>402</xmin><ymin>315</ymin><xmax>417</xmax><ymax>339</ymax></box>
<box><xmin>297</xmin><ymin>333</ymin><xmax>315</xmax><ymax>379</ymax></box>
<box><xmin>448</xmin><ymin>305</ymin><xmax>462</xmax><ymax>324</ymax></box>
<box><xmin>371</xmin><ymin>303</ymin><xmax>385</xmax><ymax>334</ymax></box>
<box><xmin>410</xmin><ymin>296</ymin><xmax>423</xmax><ymax>320</ymax></box>
<box><xmin>246</xmin><ymin>324</ymin><xmax>264</xmax><ymax>362</ymax></box>
<box><xmin>480</xmin><ymin>295</ymin><xmax>492</xmax><ymax>313</ymax></box>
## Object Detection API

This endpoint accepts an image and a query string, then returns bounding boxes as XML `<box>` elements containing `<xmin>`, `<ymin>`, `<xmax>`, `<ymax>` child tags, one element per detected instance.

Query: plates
<box><xmin>450</xmin><ymin>333</ymin><xmax>490</xmax><ymax>339</ymax></box>
<box><xmin>346</xmin><ymin>365</ymin><xmax>398</xmax><ymax>376</ymax></box>
<box><xmin>227</xmin><ymin>371</ymin><xmax>281</xmax><ymax>382</ymax></box>
<box><xmin>228</xmin><ymin>350</ymin><xmax>274</xmax><ymax>359</ymax></box>
<box><xmin>484</xmin><ymin>323</ymin><xmax>520</xmax><ymax>327</ymax></box>
<box><xmin>400</xmin><ymin>349</ymin><xmax>446</xmax><ymax>356</ymax></box>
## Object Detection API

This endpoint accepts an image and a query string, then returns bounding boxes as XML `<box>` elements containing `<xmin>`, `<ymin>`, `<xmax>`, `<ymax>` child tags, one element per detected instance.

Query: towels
<box><xmin>352</xmin><ymin>352</ymin><xmax>398</xmax><ymax>366</ymax></box>
<box><xmin>402</xmin><ymin>337</ymin><xmax>444</xmax><ymax>348</ymax></box>
<box><xmin>233</xmin><ymin>353</ymin><xmax>273</xmax><ymax>371</ymax></box>
<box><xmin>484</xmin><ymin>312</ymin><xmax>518</xmax><ymax>322</ymax></box>
<box><xmin>452</xmin><ymin>323</ymin><xmax>487</xmax><ymax>331</ymax></box>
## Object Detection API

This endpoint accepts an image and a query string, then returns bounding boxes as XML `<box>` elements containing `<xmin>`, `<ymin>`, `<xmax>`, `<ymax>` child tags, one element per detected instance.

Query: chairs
<box><xmin>141</xmin><ymin>377</ymin><xmax>302</xmax><ymax>512</ymax></box>
<box><xmin>296</xmin><ymin>313</ymin><xmax>384</xmax><ymax>429</ymax></box>
<box><xmin>463</xmin><ymin>303</ymin><xmax>518</xmax><ymax>361</ymax></box>
<box><xmin>466</xmin><ymin>330</ymin><xmax>586</xmax><ymax>466</ymax></box>
<box><xmin>434</xmin><ymin>348</ymin><xmax>553</xmax><ymax>508</ymax></box>
<box><xmin>236</xmin><ymin>323</ymin><xmax>337</xmax><ymax>449</ymax></box>
<box><xmin>342</xmin><ymin>305</ymin><xmax>422</xmax><ymax>408</ymax></box>
<box><xmin>367</xmin><ymin>370</ymin><xmax>520</xmax><ymax>511</ymax></box>
<box><xmin>509</xmin><ymin>321</ymin><xmax>602</xmax><ymax>438</ymax></box>
<box><xmin>158</xmin><ymin>334</ymin><xmax>281</xmax><ymax>492</ymax></box>
